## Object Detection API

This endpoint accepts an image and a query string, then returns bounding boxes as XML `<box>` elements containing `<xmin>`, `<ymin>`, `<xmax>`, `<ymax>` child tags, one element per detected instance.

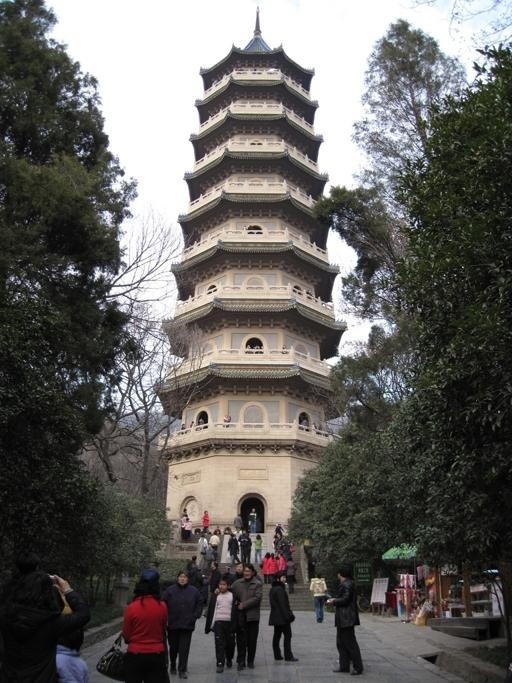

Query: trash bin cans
<box><xmin>385</xmin><ymin>591</ymin><xmax>397</xmax><ymax>615</ymax></box>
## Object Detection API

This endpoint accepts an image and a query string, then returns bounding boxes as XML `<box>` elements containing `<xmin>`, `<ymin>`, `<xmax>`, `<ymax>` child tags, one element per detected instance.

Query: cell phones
<box><xmin>47</xmin><ymin>574</ymin><xmax>59</xmax><ymax>586</ymax></box>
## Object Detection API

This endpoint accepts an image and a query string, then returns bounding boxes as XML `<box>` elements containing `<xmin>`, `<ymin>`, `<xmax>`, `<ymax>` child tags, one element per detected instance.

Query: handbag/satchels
<box><xmin>325</xmin><ymin>593</ymin><xmax>332</xmax><ymax>601</ymax></box>
<box><xmin>96</xmin><ymin>647</ymin><xmax>127</xmax><ymax>681</ymax></box>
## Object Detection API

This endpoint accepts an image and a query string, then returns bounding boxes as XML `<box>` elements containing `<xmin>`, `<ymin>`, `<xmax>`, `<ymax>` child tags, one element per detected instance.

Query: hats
<box><xmin>142</xmin><ymin>568</ymin><xmax>160</xmax><ymax>582</ymax></box>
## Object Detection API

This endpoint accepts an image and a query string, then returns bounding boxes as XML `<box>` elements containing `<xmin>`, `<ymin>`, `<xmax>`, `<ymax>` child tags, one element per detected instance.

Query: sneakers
<box><xmin>179</xmin><ymin>672</ymin><xmax>187</xmax><ymax>679</ymax></box>
<box><xmin>333</xmin><ymin>668</ymin><xmax>350</xmax><ymax>672</ymax></box>
<box><xmin>237</xmin><ymin>660</ymin><xmax>246</xmax><ymax>670</ymax></box>
<box><xmin>247</xmin><ymin>660</ymin><xmax>254</xmax><ymax>669</ymax></box>
<box><xmin>285</xmin><ymin>657</ymin><xmax>298</xmax><ymax>661</ymax></box>
<box><xmin>170</xmin><ymin>668</ymin><xmax>177</xmax><ymax>675</ymax></box>
<box><xmin>216</xmin><ymin>664</ymin><xmax>224</xmax><ymax>673</ymax></box>
<box><xmin>274</xmin><ymin>656</ymin><xmax>283</xmax><ymax>660</ymax></box>
<box><xmin>350</xmin><ymin>670</ymin><xmax>364</xmax><ymax>675</ymax></box>
<box><xmin>226</xmin><ymin>660</ymin><xmax>232</xmax><ymax>667</ymax></box>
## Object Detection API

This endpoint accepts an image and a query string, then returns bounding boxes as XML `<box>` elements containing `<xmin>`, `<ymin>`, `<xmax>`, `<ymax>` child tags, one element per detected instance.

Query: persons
<box><xmin>180</xmin><ymin>507</ymin><xmax>298</xmax><ymax>594</ymax></box>
<box><xmin>245</xmin><ymin>342</ymin><xmax>287</xmax><ymax>354</ymax></box>
<box><xmin>300</xmin><ymin>419</ymin><xmax>322</xmax><ymax>434</ymax></box>
<box><xmin>2</xmin><ymin>562</ymin><xmax>363</xmax><ymax>683</ymax></box>
<box><xmin>181</xmin><ymin>415</ymin><xmax>232</xmax><ymax>432</ymax></box>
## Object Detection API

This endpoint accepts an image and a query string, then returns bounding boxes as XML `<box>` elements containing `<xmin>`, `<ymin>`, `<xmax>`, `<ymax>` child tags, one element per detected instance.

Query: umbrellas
<box><xmin>382</xmin><ymin>543</ymin><xmax>423</xmax><ymax>588</ymax></box>
